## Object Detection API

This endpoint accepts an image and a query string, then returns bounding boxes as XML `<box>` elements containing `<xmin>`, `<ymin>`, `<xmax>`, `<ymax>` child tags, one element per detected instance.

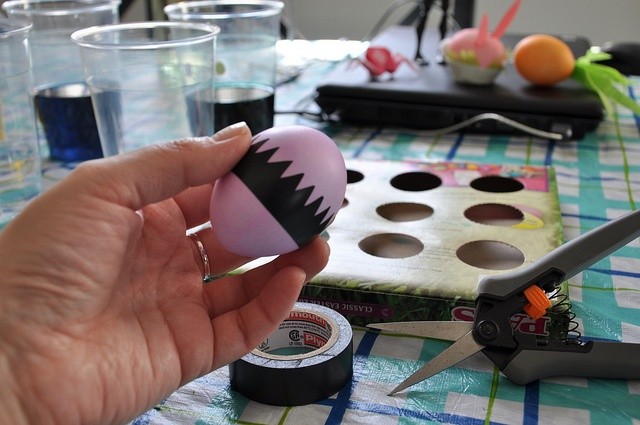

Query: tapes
<box><xmin>229</xmin><ymin>302</ymin><xmax>353</xmax><ymax>406</ymax></box>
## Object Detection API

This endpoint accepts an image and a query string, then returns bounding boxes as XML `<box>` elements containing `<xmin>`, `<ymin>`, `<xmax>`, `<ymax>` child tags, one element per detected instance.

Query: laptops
<box><xmin>314</xmin><ymin>30</ymin><xmax>605</xmax><ymax>143</ymax></box>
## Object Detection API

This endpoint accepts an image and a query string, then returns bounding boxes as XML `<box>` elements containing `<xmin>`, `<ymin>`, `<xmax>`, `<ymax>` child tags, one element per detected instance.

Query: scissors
<box><xmin>365</xmin><ymin>210</ymin><xmax>640</xmax><ymax>397</ymax></box>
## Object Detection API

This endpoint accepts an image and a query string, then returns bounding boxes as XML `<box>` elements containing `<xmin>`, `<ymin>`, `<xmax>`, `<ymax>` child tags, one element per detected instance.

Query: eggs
<box><xmin>514</xmin><ymin>34</ymin><xmax>574</xmax><ymax>86</ymax></box>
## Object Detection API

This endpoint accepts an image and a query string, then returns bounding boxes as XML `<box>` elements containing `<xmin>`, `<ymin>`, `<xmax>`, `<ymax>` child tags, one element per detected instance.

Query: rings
<box><xmin>191</xmin><ymin>231</ymin><xmax>211</xmax><ymax>281</ymax></box>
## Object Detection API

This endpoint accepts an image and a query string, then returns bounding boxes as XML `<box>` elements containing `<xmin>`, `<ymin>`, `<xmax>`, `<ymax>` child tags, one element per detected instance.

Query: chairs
<box><xmin>111</xmin><ymin>1</ymin><xmax>289</xmax><ymax>41</ymax></box>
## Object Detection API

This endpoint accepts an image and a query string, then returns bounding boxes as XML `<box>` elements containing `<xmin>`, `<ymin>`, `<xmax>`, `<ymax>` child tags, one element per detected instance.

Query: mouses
<box><xmin>595</xmin><ymin>38</ymin><xmax>640</xmax><ymax>76</ymax></box>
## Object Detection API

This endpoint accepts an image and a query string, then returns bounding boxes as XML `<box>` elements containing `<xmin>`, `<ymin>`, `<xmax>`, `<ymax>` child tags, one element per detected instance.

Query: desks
<box><xmin>1</xmin><ymin>38</ymin><xmax>640</xmax><ymax>424</ymax></box>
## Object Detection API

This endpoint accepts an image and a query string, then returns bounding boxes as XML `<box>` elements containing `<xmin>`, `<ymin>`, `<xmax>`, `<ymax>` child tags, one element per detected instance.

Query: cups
<box><xmin>70</xmin><ymin>21</ymin><xmax>221</xmax><ymax>159</ymax></box>
<box><xmin>164</xmin><ymin>1</ymin><xmax>286</xmax><ymax>137</ymax></box>
<box><xmin>1</xmin><ymin>18</ymin><xmax>42</xmax><ymax>212</ymax></box>
<box><xmin>1</xmin><ymin>0</ymin><xmax>123</xmax><ymax>169</ymax></box>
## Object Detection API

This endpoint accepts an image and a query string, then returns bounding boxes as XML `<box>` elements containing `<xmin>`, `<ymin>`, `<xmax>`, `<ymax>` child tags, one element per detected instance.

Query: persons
<box><xmin>1</xmin><ymin>119</ymin><xmax>332</xmax><ymax>423</ymax></box>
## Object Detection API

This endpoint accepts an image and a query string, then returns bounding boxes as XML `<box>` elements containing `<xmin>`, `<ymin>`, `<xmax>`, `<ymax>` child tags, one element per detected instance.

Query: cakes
<box><xmin>439</xmin><ymin>28</ymin><xmax>505</xmax><ymax>84</ymax></box>
